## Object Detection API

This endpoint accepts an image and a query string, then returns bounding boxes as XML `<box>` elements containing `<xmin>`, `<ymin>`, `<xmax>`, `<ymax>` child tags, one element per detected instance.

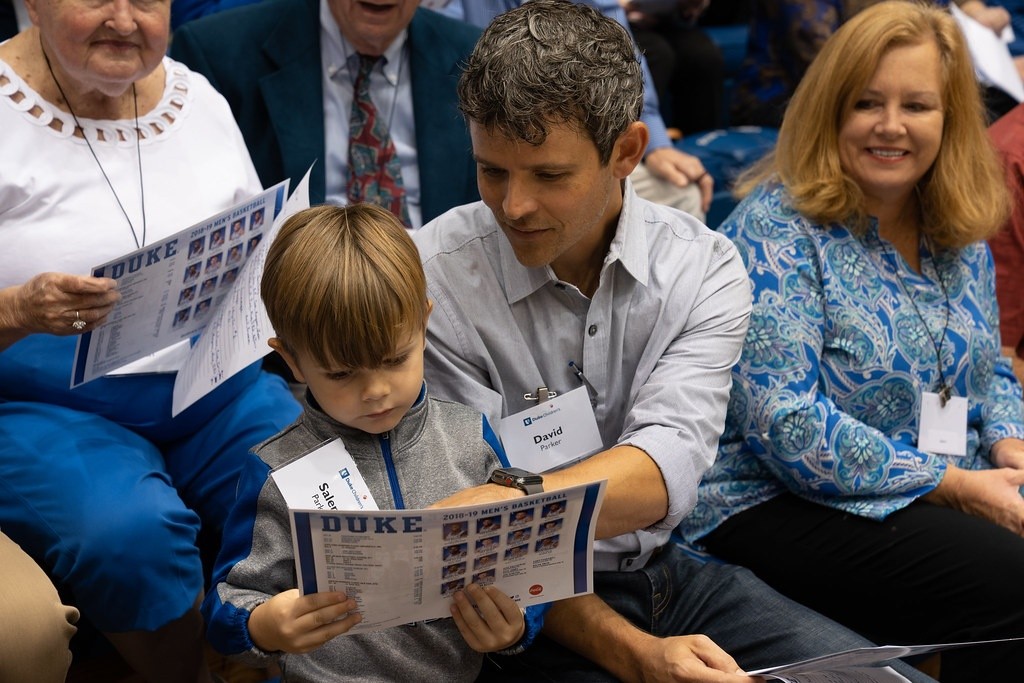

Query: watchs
<box><xmin>485</xmin><ymin>466</ymin><xmax>545</xmax><ymax>498</ymax></box>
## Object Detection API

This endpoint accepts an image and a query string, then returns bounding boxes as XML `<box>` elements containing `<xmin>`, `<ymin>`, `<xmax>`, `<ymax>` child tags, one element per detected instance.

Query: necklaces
<box><xmin>37</xmin><ymin>28</ymin><xmax>147</xmax><ymax>250</ymax></box>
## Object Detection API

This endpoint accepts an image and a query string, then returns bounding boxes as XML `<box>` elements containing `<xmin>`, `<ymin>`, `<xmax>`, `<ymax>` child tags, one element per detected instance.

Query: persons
<box><xmin>442</xmin><ymin>501</ymin><xmax>568</xmax><ymax>597</ymax></box>
<box><xmin>169</xmin><ymin>0</ymin><xmax>1024</xmax><ymax>385</ymax></box>
<box><xmin>174</xmin><ymin>207</ymin><xmax>264</xmax><ymax>327</ymax></box>
<box><xmin>674</xmin><ymin>0</ymin><xmax>1024</xmax><ymax>683</ymax></box>
<box><xmin>200</xmin><ymin>205</ymin><xmax>553</xmax><ymax>683</ymax></box>
<box><xmin>0</xmin><ymin>0</ymin><xmax>303</xmax><ymax>683</ymax></box>
<box><xmin>409</xmin><ymin>0</ymin><xmax>938</xmax><ymax>683</ymax></box>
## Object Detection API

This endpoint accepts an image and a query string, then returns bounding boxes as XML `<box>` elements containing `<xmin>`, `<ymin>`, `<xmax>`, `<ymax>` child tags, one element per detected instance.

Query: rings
<box><xmin>71</xmin><ymin>309</ymin><xmax>87</xmax><ymax>334</ymax></box>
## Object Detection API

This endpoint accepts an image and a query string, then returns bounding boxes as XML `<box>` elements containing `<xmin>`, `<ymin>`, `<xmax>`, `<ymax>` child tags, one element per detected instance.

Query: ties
<box><xmin>346</xmin><ymin>51</ymin><xmax>413</xmax><ymax>230</ymax></box>
